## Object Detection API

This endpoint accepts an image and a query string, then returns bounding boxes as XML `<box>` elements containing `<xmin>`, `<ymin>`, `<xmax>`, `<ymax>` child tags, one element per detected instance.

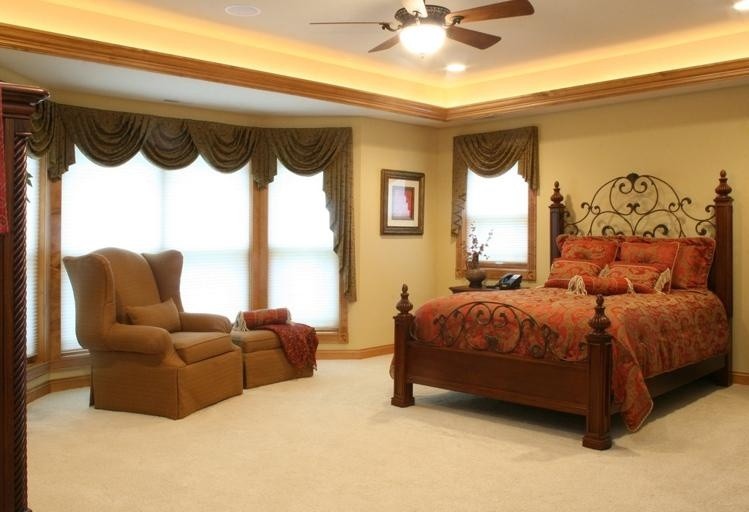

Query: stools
<box><xmin>231</xmin><ymin>322</ymin><xmax>313</xmax><ymax>389</ymax></box>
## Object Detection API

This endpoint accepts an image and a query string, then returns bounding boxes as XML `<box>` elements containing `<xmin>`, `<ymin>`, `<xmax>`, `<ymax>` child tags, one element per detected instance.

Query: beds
<box><xmin>390</xmin><ymin>169</ymin><xmax>733</xmax><ymax>450</ymax></box>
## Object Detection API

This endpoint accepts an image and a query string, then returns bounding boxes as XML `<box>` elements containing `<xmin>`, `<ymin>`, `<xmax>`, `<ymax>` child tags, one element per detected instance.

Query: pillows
<box><xmin>598</xmin><ymin>262</ymin><xmax>671</xmax><ymax>296</ymax></box>
<box><xmin>555</xmin><ymin>232</ymin><xmax>618</xmax><ymax>268</ymax></box>
<box><xmin>545</xmin><ymin>258</ymin><xmax>601</xmax><ymax>288</ymax></box>
<box><xmin>621</xmin><ymin>241</ymin><xmax>679</xmax><ymax>295</ymax></box>
<box><xmin>567</xmin><ymin>276</ymin><xmax>636</xmax><ymax>300</ymax></box>
<box><xmin>231</xmin><ymin>308</ymin><xmax>292</xmax><ymax>335</ymax></box>
<box><xmin>620</xmin><ymin>237</ymin><xmax>716</xmax><ymax>295</ymax></box>
<box><xmin>124</xmin><ymin>297</ymin><xmax>182</xmax><ymax>333</ymax></box>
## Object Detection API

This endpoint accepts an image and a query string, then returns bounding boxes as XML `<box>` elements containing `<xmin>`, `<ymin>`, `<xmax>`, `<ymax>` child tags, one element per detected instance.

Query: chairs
<box><xmin>63</xmin><ymin>247</ymin><xmax>242</xmax><ymax>419</ymax></box>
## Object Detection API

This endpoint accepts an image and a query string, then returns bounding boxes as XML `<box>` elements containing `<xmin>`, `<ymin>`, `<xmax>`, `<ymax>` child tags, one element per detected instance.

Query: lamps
<box><xmin>397</xmin><ymin>23</ymin><xmax>448</xmax><ymax>60</ymax></box>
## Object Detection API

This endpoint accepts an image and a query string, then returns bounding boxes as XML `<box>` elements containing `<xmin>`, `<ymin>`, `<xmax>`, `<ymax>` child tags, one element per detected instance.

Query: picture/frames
<box><xmin>380</xmin><ymin>169</ymin><xmax>425</xmax><ymax>235</ymax></box>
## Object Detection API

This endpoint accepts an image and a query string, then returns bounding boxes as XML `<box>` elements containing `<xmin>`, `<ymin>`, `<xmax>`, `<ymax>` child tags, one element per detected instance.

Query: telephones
<box><xmin>497</xmin><ymin>272</ymin><xmax>522</xmax><ymax>289</ymax></box>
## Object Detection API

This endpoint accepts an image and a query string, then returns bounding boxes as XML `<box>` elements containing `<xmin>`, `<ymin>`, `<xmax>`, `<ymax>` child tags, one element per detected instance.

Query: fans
<box><xmin>308</xmin><ymin>0</ymin><xmax>535</xmax><ymax>60</ymax></box>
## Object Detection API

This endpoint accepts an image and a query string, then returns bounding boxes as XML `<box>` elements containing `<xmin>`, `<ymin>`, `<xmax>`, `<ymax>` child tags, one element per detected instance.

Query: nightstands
<box><xmin>449</xmin><ymin>284</ymin><xmax>529</xmax><ymax>294</ymax></box>
